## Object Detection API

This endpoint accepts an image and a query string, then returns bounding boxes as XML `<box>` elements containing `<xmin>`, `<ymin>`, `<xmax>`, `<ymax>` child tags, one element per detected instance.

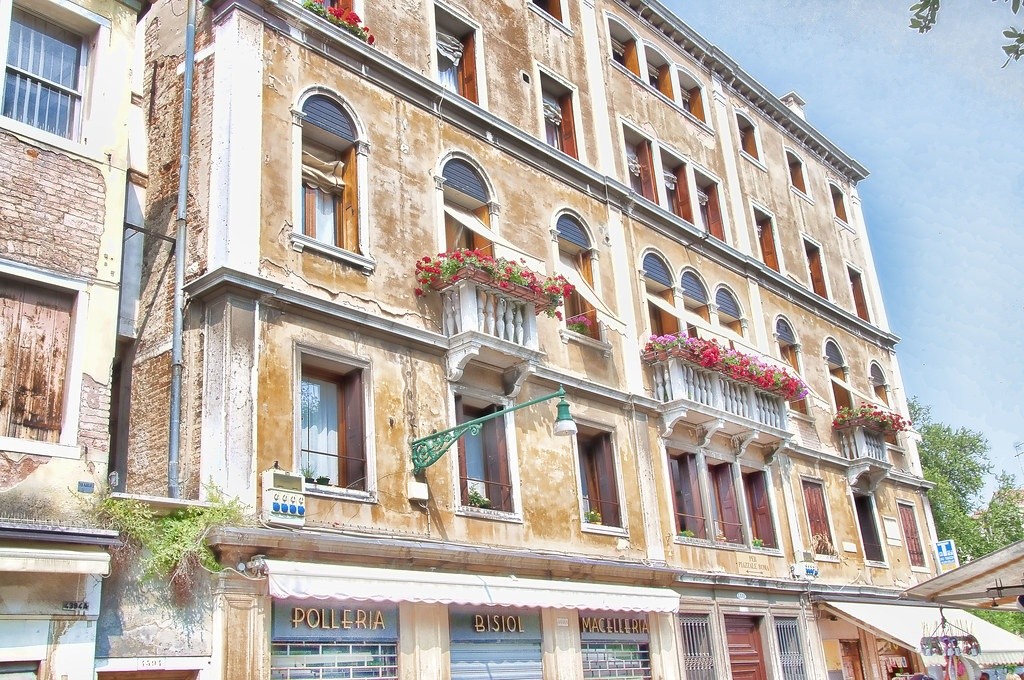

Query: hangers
<box><xmin>931</xmin><ymin>607</ymin><xmax>970</xmax><ymax>637</ymax></box>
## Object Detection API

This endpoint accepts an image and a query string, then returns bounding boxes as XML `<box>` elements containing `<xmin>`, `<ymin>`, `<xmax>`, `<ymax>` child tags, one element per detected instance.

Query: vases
<box><xmin>534</xmin><ymin>292</ymin><xmax>550</xmax><ymax>305</ymax></box>
<box><xmin>849</xmin><ymin>419</ymin><xmax>861</xmax><ymax>426</ymax></box>
<box><xmin>431</xmin><ymin>278</ymin><xmax>447</xmax><ymax>289</ymax></box>
<box><xmin>834</xmin><ymin>421</ymin><xmax>849</xmax><ymax>430</ymax></box>
<box><xmin>511</xmin><ymin>284</ymin><xmax>528</xmax><ymax>296</ymax></box>
<box><xmin>640</xmin><ymin>343</ymin><xmax>775</xmax><ymax>403</ymax></box>
<box><xmin>491</xmin><ymin>274</ymin><xmax>515</xmax><ymax>292</ymax></box>
<box><xmin>470</xmin><ymin>268</ymin><xmax>489</xmax><ymax>283</ymax></box>
<box><xmin>523</xmin><ymin>287</ymin><xmax>539</xmax><ymax>301</ymax></box>
<box><xmin>591</xmin><ymin>522</ymin><xmax>601</xmax><ymax>525</ymax></box>
<box><xmin>456</xmin><ymin>266</ymin><xmax>474</xmax><ymax>279</ymax></box>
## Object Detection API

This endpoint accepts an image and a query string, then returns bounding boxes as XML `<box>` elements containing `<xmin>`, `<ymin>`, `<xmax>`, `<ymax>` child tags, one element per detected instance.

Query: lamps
<box><xmin>411</xmin><ymin>384</ymin><xmax>577</xmax><ymax>476</ymax></box>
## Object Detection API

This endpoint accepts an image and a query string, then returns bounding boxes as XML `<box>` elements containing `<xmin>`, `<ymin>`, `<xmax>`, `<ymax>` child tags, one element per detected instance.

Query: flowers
<box><xmin>645</xmin><ymin>333</ymin><xmax>808</xmax><ymax>402</ymax></box>
<box><xmin>415</xmin><ymin>248</ymin><xmax>575</xmax><ymax>321</ymax></box>
<box><xmin>566</xmin><ymin>316</ymin><xmax>591</xmax><ymax>334</ymax></box>
<box><xmin>832</xmin><ymin>402</ymin><xmax>912</xmax><ymax>431</ymax></box>
<box><xmin>302</xmin><ymin>0</ymin><xmax>374</xmax><ymax>45</ymax></box>
<box><xmin>585</xmin><ymin>507</ymin><xmax>601</xmax><ymax>522</ymax></box>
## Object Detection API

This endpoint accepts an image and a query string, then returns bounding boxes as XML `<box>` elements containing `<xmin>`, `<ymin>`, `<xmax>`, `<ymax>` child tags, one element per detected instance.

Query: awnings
<box><xmin>264</xmin><ymin>560</ymin><xmax>681</xmax><ymax>615</ymax></box>
<box><xmin>818</xmin><ymin>602</ymin><xmax>1024</xmax><ymax>665</ymax></box>
<box><xmin>899</xmin><ymin>538</ymin><xmax>1024</xmax><ymax>611</ymax></box>
